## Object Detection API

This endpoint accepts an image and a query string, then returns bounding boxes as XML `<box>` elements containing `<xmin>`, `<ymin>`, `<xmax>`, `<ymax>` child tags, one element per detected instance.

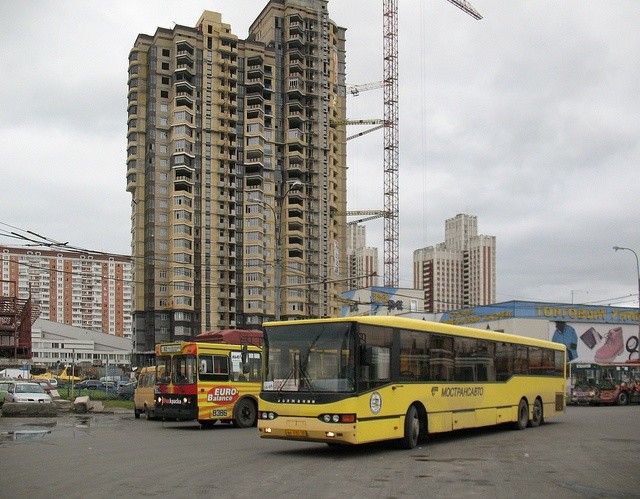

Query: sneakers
<box><xmin>594</xmin><ymin>326</ymin><xmax>624</xmax><ymax>364</ymax></box>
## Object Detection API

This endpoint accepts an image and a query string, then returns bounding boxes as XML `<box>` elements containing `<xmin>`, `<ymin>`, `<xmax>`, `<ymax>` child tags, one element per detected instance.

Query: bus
<box><xmin>569</xmin><ymin>361</ymin><xmax>639</xmax><ymax>406</ymax></box>
<box><xmin>257</xmin><ymin>315</ymin><xmax>567</xmax><ymax>449</ymax></box>
<box><xmin>154</xmin><ymin>340</ymin><xmax>350</xmax><ymax>427</ymax></box>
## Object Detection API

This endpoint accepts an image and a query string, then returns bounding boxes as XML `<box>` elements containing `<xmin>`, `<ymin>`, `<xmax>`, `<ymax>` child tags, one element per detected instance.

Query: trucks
<box><xmin>0</xmin><ymin>368</ymin><xmax>30</xmax><ymax>380</ymax></box>
<box><xmin>192</xmin><ymin>330</ymin><xmax>266</xmax><ymax>346</ymax></box>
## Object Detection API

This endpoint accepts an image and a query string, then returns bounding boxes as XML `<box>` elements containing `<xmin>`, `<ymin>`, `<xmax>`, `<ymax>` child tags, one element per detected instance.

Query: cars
<box><xmin>73</xmin><ymin>379</ymin><xmax>116</xmax><ymax>391</ymax></box>
<box><xmin>0</xmin><ymin>382</ymin><xmax>14</xmax><ymax>404</ymax></box>
<box><xmin>5</xmin><ymin>382</ymin><xmax>52</xmax><ymax>405</ymax></box>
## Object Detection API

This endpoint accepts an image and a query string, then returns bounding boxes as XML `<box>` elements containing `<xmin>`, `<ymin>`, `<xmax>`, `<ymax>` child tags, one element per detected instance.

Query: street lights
<box><xmin>612</xmin><ymin>245</ymin><xmax>640</xmax><ymax>310</ymax></box>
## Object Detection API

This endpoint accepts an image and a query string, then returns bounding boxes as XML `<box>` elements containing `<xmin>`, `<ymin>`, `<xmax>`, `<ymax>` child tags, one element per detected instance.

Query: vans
<box><xmin>48</xmin><ymin>379</ymin><xmax>59</xmax><ymax>388</ymax></box>
<box><xmin>133</xmin><ymin>364</ymin><xmax>196</xmax><ymax>419</ymax></box>
